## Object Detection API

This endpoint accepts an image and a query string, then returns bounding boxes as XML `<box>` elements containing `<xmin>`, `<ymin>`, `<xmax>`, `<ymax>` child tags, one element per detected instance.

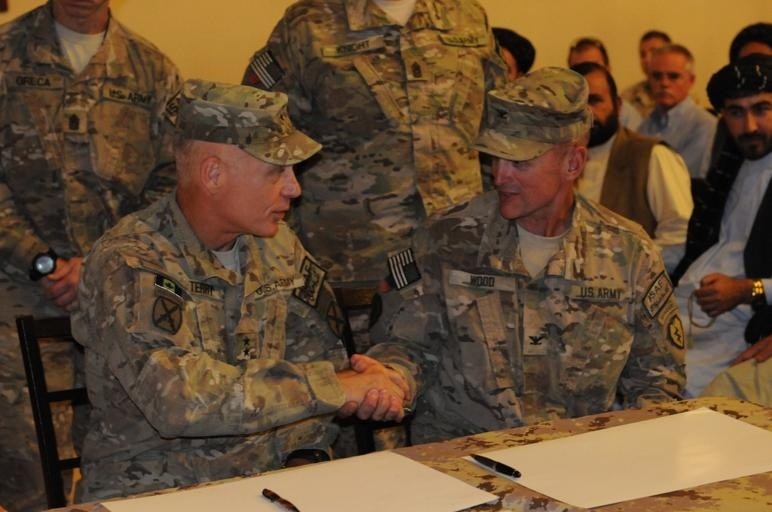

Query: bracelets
<box><xmin>687</xmin><ymin>287</ymin><xmax>715</xmax><ymax>328</ymax></box>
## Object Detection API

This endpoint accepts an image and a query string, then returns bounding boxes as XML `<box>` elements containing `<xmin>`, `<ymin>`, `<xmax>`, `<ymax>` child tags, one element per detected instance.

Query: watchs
<box><xmin>29</xmin><ymin>248</ymin><xmax>57</xmax><ymax>282</ymax></box>
<box><xmin>750</xmin><ymin>278</ymin><xmax>766</xmax><ymax>306</ymax></box>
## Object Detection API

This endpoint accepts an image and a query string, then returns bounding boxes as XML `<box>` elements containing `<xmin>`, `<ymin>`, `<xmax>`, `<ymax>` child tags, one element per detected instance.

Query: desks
<box><xmin>34</xmin><ymin>394</ymin><xmax>772</xmax><ymax>512</ymax></box>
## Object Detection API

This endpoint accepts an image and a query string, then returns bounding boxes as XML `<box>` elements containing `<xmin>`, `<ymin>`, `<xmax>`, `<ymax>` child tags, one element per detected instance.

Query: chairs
<box><xmin>15</xmin><ymin>315</ymin><xmax>88</xmax><ymax>510</ymax></box>
<box><xmin>332</xmin><ymin>288</ymin><xmax>412</xmax><ymax>454</ymax></box>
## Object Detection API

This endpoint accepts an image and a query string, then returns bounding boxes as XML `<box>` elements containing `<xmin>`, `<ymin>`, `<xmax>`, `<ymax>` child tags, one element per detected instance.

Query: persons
<box><xmin>242</xmin><ymin>0</ymin><xmax>511</xmax><ymax>460</ymax></box>
<box><xmin>72</xmin><ymin>79</ymin><xmax>411</xmax><ymax>503</ymax></box>
<box><xmin>717</xmin><ymin>21</ymin><xmax>771</xmax><ymax>56</ymax></box>
<box><xmin>567</xmin><ymin>36</ymin><xmax>614</xmax><ymax>72</ymax></box>
<box><xmin>654</xmin><ymin>54</ymin><xmax>771</xmax><ymax>399</ymax></box>
<box><xmin>698</xmin><ymin>333</ymin><xmax>771</xmax><ymax>408</ymax></box>
<box><xmin>633</xmin><ymin>44</ymin><xmax>718</xmax><ymax>180</ymax></box>
<box><xmin>569</xmin><ymin>62</ymin><xmax>694</xmax><ymax>247</ymax></box>
<box><xmin>617</xmin><ymin>32</ymin><xmax>709</xmax><ymax>135</ymax></box>
<box><xmin>490</xmin><ymin>27</ymin><xmax>537</xmax><ymax>85</ymax></box>
<box><xmin>336</xmin><ymin>66</ymin><xmax>687</xmax><ymax>444</ymax></box>
<box><xmin>2</xmin><ymin>0</ymin><xmax>182</xmax><ymax>512</ymax></box>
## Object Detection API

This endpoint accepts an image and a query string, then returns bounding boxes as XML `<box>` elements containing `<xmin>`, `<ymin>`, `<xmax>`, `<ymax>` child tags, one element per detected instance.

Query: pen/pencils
<box><xmin>470</xmin><ymin>453</ymin><xmax>521</xmax><ymax>478</ymax></box>
<box><xmin>262</xmin><ymin>489</ymin><xmax>300</xmax><ymax>512</ymax></box>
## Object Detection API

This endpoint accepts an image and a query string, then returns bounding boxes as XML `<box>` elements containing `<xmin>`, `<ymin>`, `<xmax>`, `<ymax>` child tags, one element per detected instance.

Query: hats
<box><xmin>467</xmin><ymin>66</ymin><xmax>592</xmax><ymax>161</ymax></box>
<box><xmin>176</xmin><ymin>79</ymin><xmax>324</xmax><ymax>166</ymax></box>
